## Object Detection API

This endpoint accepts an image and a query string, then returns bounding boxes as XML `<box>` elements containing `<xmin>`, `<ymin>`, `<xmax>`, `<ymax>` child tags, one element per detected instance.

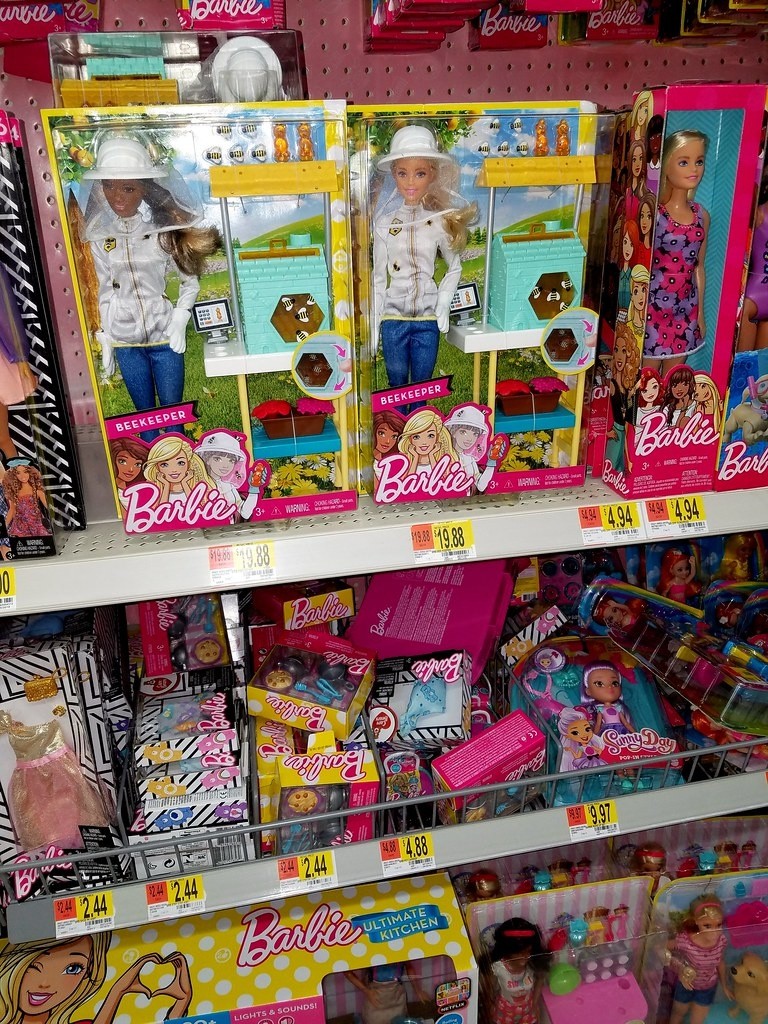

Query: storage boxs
<box><xmin>495</xmin><ymin>598</ymin><xmax>567</xmax><ymax>666</ymax></box>
<box><xmin>250</xmin><ymin>578</ymin><xmax>357</xmax><ymax>631</ymax></box>
<box><xmin>142</xmin><ymin>788</ymin><xmax>249</xmax><ymax>833</ymax></box>
<box><xmin>254</xmin><ymin>717</ymin><xmax>296</xmax><ymax>853</ymax></box>
<box><xmin>269</xmin><ymin>749</ymin><xmax>380</xmax><ymax>856</ymax></box>
<box><xmin>246</xmin><ymin>644</ymin><xmax>375</xmax><ymax>742</ymax></box>
<box><xmin>135</xmin><ymin>750</ymin><xmax>241</xmax><ymax>802</ymax></box>
<box><xmin>127</xmin><ymin>807</ymin><xmax>251</xmax><ymax>858</ymax></box>
<box><xmin>133</xmin><ymin>689</ymin><xmax>241</xmax><ymax>768</ymax></box>
<box><xmin>348</xmin><ymin>102</ymin><xmax>598</xmax><ymax>504</ymax></box>
<box><xmin>0</xmin><ymin>816</ymin><xmax>768</xmax><ymax>1024</ymax></box>
<box><xmin>0</xmin><ymin>635</ymin><xmax>132</xmax><ymax>899</ymax></box>
<box><xmin>369</xmin><ymin>647</ymin><xmax>472</xmax><ymax>749</ymax></box>
<box><xmin>430</xmin><ymin>709</ymin><xmax>547</xmax><ymax>826</ymax></box>
<box><xmin>600</xmin><ymin>79</ymin><xmax>768</xmax><ymax>500</ymax></box>
<box><xmin>39</xmin><ymin>99</ymin><xmax>359</xmax><ymax>535</ymax></box>
<box><xmin>0</xmin><ymin>0</ymin><xmax>768</xmax><ymax>108</ymax></box>
<box><xmin>76</xmin><ymin>636</ymin><xmax>129</xmax><ymax>848</ymax></box>
<box><xmin>138</xmin><ymin>593</ymin><xmax>245</xmax><ymax>679</ymax></box>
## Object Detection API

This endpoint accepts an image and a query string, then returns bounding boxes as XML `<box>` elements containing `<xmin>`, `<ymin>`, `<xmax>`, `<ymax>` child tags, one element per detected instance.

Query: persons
<box><xmin>0</xmin><ymin>125</ymin><xmax>768</xmax><ymax>1024</ymax></box>
<box><xmin>80</xmin><ymin>138</ymin><xmax>220</xmax><ymax>445</ymax></box>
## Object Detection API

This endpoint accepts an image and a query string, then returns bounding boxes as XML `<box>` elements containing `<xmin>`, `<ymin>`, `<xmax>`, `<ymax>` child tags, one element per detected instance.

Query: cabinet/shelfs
<box><xmin>0</xmin><ymin>0</ymin><xmax>767</xmax><ymax>947</ymax></box>
